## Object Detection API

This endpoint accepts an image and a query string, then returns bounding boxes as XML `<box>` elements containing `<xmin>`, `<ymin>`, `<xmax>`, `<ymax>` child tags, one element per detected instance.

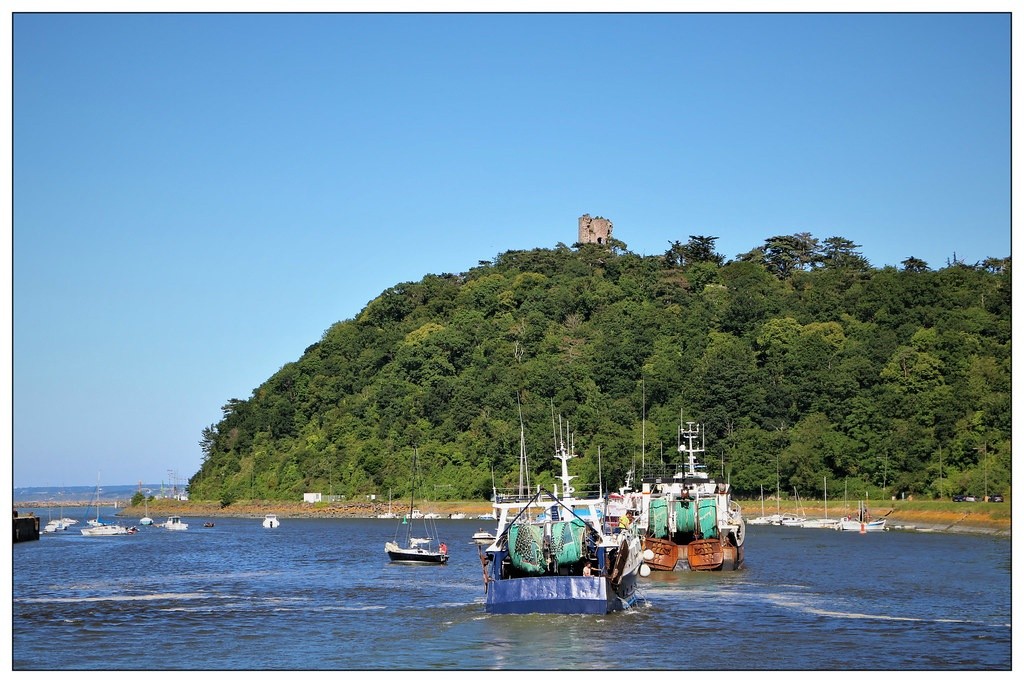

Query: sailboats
<box><xmin>385</xmin><ymin>449</ymin><xmax>450</xmax><ymax>565</ymax></box>
<box><xmin>747</xmin><ymin>484</ymin><xmax>807</xmax><ymax>527</ymax></box>
<box><xmin>802</xmin><ymin>476</ymin><xmax>841</xmax><ymax>529</ymax></box>
<box><xmin>80</xmin><ymin>472</ymin><xmax>128</xmax><ymax>536</ymax></box>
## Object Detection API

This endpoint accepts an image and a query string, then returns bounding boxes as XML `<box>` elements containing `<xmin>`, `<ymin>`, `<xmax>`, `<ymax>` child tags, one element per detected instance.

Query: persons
<box><xmin>440</xmin><ymin>542</ymin><xmax>447</xmax><ymax>554</ymax></box>
<box><xmin>619</xmin><ymin>511</ymin><xmax>631</xmax><ymax>528</ymax></box>
<box><xmin>583</xmin><ymin>561</ymin><xmax>601</xmax><ymax>577</ymax></box>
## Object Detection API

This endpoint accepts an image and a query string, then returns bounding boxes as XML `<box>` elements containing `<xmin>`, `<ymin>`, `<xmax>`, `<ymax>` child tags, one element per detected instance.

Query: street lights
<box><xmin>877</xmin><ymin>454</ymin><xmax>887</xmax><ymax>500</ymax></box>
<box><xmin>973</xmin><ymin>441</ymin><xmax>988</xmax><ymax>502</ymax></box>
<box><xmin>931</xmin><ymin>446</ymin><xmax>942</xmax><ymax>498</ymax></box>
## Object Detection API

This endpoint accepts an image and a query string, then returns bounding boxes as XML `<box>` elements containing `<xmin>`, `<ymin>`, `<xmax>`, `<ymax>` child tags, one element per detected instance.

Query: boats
<box><xmin>161</xmin><ymin>516</ymin><xmax>188</xmax><ymax>530</ymax></box>
<box><xmin>139</xmin><ymin>518</ymin><xmax>154</xmax><ymax>525</ymax></box>
<box><xmin>45</xmin><ymin>518</ymin><xmax>79</xmax><ymax>532</ymax></box>
<box><xmin>600</xmin><ymin>422</ymin><xmax>745</xmax><ymax>571</ymax></box>
<box><xmin>127</xmin><ymin>527</ymin><xmax>140</xmax><ymax>534</ymax></box>
<box><xmin>263</xmin><ymin>514</ymin><xmax>280</xmax><ymax>529</ymax></box>
<box><xmin>86</xmin><ymin>519</ymin><xmax>97</xmax><ymax>525</ymax></box>
<box><xmin>472</xmin><ymin>533</ymin><xmax>496</xmax><ymax>545</ymax></box>
<box><xmin>840</xmin><ymin>501</ymin><xmax>886</xmax><ymax>531</ymax></box>
<box><xmin>203</xmin><ymin>522</ymin><xmax>214</xmax><ymax>527</ymax></box>
<box><xmin>480</xmin><ymin>414</ymin><xmax>644</xmax><ymax>615</ymax></box>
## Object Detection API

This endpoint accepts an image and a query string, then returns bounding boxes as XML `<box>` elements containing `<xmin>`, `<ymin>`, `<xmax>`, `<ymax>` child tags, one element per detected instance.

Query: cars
<box><xmin>952</xmin><ymin>495</ymin><xmax>966</xmax><ymax>502</ymax></box>
<box><xmin>988</xmin><ymin>494</ymin><xmax>1004</xmax><ymax>502</ymax></box>
<box><xmin>966</xmin><ymin>495</ymin><xmax>982</xmax><ymax>502</ymax></box>
